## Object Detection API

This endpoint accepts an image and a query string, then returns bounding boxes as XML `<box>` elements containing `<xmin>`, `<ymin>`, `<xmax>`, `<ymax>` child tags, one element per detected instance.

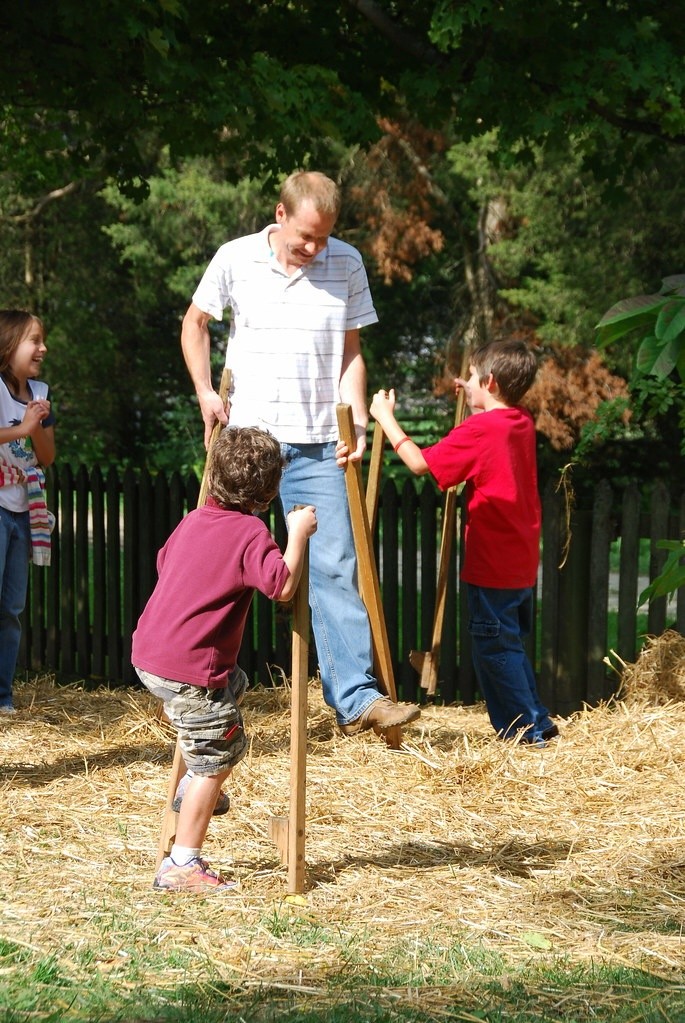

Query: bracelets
<box><xmin>394</xmin><ymin>437</ymin><xmax>410</xmax><ymax>452</ymax></box>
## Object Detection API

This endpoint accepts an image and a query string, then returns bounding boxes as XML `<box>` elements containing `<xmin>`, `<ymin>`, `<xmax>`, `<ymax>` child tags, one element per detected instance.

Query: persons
<box><xmin>131</xmin><ymin>425</ymin><xmax>317</xmax><ymax>893</ymax></box>
<box><xmin>369</xmin><ymin>340</ymin><xmax>558</xmax><ymax>745</ymax></box>
<box><xmin>0</xmin><ymin>309</ymin><xmax>54</xmax><ymax>712</ymax></box>
<box><xmin>181</xmin><ymin>171</ymin><xmax>422</xmax><ymax>735</ymax></box>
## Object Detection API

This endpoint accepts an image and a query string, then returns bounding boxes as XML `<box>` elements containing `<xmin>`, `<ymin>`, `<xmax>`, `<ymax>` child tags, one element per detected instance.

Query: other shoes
<box><xmin>0</xmin><ymin>705</ymin><xmax>16</xmax><ymax>714</ymax></box>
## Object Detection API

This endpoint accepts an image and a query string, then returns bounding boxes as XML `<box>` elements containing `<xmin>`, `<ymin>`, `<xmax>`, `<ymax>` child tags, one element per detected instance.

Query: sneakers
<box><xmin>172</xmin><ymin>772</ymin><xmax>230</xmax><ymax>815</ymax></box>
<box><xmin>152</xmin><ymin>857</ymin><xmax>238</xmax><ymax>894</ymax></box>
<box><xmin>338</xmin><ymin>695</ymin><xmax>421</xmax><ymax>737</ymax></box>
